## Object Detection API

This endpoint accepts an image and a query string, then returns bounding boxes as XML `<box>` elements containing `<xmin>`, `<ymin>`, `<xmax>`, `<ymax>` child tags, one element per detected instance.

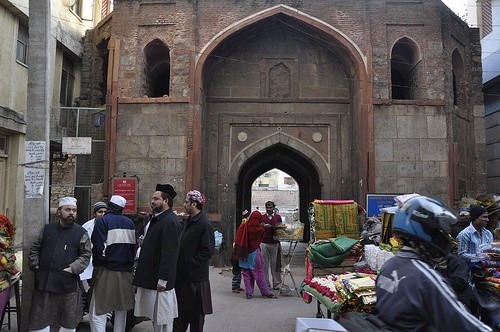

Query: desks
<box><xmin>273</xmin><ymin>236</ymin><xmax>303</xmax><ymax>299</ymax></box>
<box><xmin>304</xmin><ymin>275</ymin><xmax>378</xmax><ymax>320</ymax></box>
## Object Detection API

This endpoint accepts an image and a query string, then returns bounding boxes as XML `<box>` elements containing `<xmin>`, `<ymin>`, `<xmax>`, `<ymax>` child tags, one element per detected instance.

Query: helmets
<box><xmin>392</xmin><ymin>196</ymin><xmax>459</xmax><ymax>256</ymax></box>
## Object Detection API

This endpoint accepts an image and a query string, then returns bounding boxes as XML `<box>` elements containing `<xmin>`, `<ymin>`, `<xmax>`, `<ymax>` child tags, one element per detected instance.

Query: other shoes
<box><xmin>262</xmin><ymin>294</ymin><xmax>278</xmax><ymax>299</ymax></box>
<box><xmin>246</xmin><ymin>294</ymin><xmax>252</xmax><ymax>299</ymax></box>
<box><xmin>233</xmin><ymin>288</ymin><xmax>244</xmax><ymax>293</ymax></box>
<box><xmin>273</xmin><ymin>284</ymin><xmax>290</xmax><ymax>291</ymax></box>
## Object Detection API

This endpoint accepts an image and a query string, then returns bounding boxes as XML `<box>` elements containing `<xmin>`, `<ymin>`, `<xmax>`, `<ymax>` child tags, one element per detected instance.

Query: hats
<box><xmin>58</xmin><ymin>197</ymin><xmax>77</xmax><ymax>207</ymax></box>
<box><xmin>93</xmin><ymin>201</ymin><xmax>108</xmax><ymax>213</ymax></box>
<box><xmin>469</xmin><ymin>203</ymin><xmax>487</xmax><ymax>224</ymax></box>
<box><xmin>156</xmin><ymin>183</ymin><xmax>177</xmax><ymax>199</ymax></box>
<box><xmin>241</xmin><ymin>209</ymin><xmax>250</xmax><ymax>218</ymax></box>
<box><xmin>109</xmin><ymin>195</ymin><xmax>126</xmax><ymax>208</ymax></box>
<box><xmin>187</xmin><ymin>189</ymin><xmax>207</xmax><ymax>208</ymax></box>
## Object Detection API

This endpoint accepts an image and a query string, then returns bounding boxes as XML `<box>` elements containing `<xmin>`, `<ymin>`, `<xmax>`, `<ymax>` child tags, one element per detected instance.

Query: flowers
<box><xmin>0</xmin><ymin>213</ymin><xmax>19</xmax><ymax>287</ymax></box>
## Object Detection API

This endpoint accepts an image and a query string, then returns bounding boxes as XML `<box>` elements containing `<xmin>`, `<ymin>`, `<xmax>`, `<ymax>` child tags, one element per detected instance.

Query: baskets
<box><xmin>294</xmin><ymin>317</ymin><xmax>348</xmax><ymax>332</ymax></box>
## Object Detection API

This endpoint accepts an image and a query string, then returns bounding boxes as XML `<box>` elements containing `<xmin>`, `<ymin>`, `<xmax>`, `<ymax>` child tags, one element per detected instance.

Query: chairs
<box><xmin>302</xmin><ymin>199</ymin><xmax>377</xmax><ymax>320</ymax></box>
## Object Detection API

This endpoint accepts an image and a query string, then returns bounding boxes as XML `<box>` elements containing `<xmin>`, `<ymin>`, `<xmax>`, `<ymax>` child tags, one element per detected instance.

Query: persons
<box><xmin>259</xmin><ymin>202</ymin><xmax>288</xmax><ymax>290</ymax></box>
<box><xmin>89</xmin><ymin>195</ymin><xmax>138</xmax><ymax>332</ymax></box>
<box><xmin>230</xmin><ymin>209</ymin><xmax>250</xmax><ymax>294</ymax></box>
<box><xmin>28</xmin><ymin>197</ymin><xmax>91</xmax><ymax>332</ymax></box>
<box><xmin>77</xmin><ymin>201</ymin><xmax>108</xmax><ymax>315</ymax></box>
<box><xmin>133</xmin><ymin>184</ymin><xmax>180</xmax><ymax>332</ymax></box>
<box><xmin>375</xmin><ymin>197</ymin><xmax>500</xmax><ymax>332</ymax></box>
<box><xmin>233</xmin><ymin>211</ymin><xmax>276</xmax><ymax>299</ymax></box>
<box><xmin>0</xmin><ymin>214</ymin><xmax>16</xmax><ymax>332</ymax></box>
<box><xmin>173</xmin><ymin>189</ymin><xmax>215</xmax><ymax>332</ymax></box>
